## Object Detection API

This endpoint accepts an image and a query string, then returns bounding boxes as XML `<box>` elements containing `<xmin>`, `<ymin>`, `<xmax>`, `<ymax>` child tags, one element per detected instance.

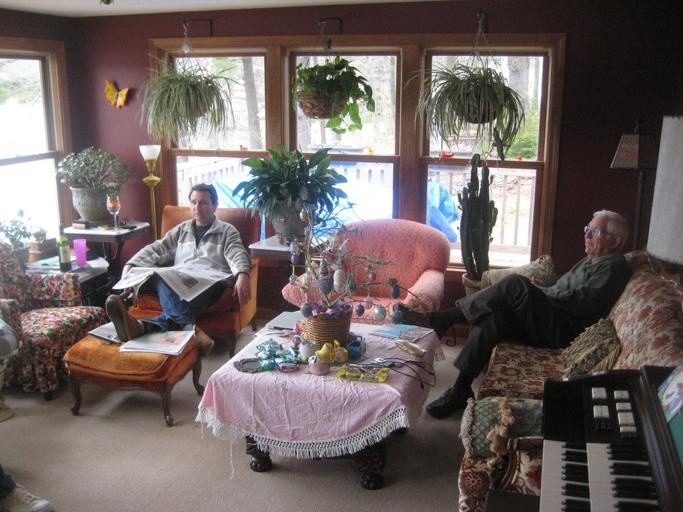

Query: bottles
<box><xmin>55</xmin><ymin>224</ymin><xmax>70</xmax><ymax>271</ymax></box>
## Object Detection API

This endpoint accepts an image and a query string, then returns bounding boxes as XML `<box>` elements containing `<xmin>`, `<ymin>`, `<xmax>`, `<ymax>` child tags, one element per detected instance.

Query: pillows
<box><xmin>481</xmin><ymin>253</ymin><xmax>557</xmax><ymax>292</ymax></box>
<box><xmin>559</xmin><ymin>318</ymin><xmax>621</xmax><ymax>380</ymax></box>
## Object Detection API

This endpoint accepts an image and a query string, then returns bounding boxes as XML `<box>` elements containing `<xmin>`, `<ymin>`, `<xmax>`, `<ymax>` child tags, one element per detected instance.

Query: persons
<box><xmin>105</xmin><ymin>183</ymin><xmax>251</xmax><ymax>357</ymax></box>
<box><xmin>392</xmin><ymin>210</ymin><xmax>629</xmax><ymax>419</ymax></box>
<box><xmin>0</xmin><ymin>463</ymin><xmax>52</xmax><ymax>512</ymax></box>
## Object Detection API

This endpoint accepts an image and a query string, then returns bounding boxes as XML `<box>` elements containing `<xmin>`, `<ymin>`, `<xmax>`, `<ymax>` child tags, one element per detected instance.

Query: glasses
<box><xmin>584</xmin><ymin>226</ymin><xmax>610</xmax><ymax>238</ymax></box>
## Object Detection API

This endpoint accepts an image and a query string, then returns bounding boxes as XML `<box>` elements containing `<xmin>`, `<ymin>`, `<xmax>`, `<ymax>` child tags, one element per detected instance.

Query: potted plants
<box><xmin>454</xmin><ymin>152</ymin><xmax>498</xmax><ymax>298</ymax></box>
<box><xmin>289</xmin><ymin>53</ymin><xmax>376</xmax><ymax>136</ymax></box>
<box><xmin>0</xmin><ymin>209</ymin><xmax>48</xmax><ymax>271</ymax></box>
<box><xmin>230</xmin><ymin>144</ymin><xmax>348</xmax><ymax>237</ymax></box>
<box><xmin>132</xmin><ymin>51</ymin><xmax>242</xmax><ymax>155</ymax></box>
<box><xmin>56</xmin><ymin>143</ymin><xmax>133</xmax><ymax>226</ymax></box>
<box><xmin>401</xmin><ymin>47</ymin><xmax>526</xmax><ymax>166</ymax></box>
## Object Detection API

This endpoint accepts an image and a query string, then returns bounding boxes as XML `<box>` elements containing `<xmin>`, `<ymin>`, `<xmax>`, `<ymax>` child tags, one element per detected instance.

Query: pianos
<box><xmin>535</xmin><ymin>363</ymin><xmax>681</xmax><ymax>506</ymax></box>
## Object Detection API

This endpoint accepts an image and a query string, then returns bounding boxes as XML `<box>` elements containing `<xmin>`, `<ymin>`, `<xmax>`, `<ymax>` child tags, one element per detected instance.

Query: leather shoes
<box><xmin>392</xmin><ymin>304</ymin><xmax>443</xmax><ymax>337</ymax></box>
<box><xmin>426</xmin><ymin>385</ymin><xmax>473</xmax><ymax>417</ymax></box>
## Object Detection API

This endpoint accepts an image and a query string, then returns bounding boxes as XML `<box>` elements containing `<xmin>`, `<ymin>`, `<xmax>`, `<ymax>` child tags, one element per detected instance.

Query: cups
<box><xmin>72</xmin><ymin>239</ymin><xmax>87</xmax><ymax>266</ymax></box>
<box><xmin>299</xmin><ymin>340</ymin><xmax>315</xmax><ymax>358</ymax></box>
<box><xmin>440</xmin><ymin>136</ymin><xmax>454</xmax><ymax>157</ymax></box>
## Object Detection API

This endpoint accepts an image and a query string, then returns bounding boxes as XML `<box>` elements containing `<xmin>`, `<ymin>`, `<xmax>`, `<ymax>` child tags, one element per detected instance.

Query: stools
<box><xmin>481</xmin><ymin>489</ymin><xmax>540</xmax><ymax>511</ymax></box>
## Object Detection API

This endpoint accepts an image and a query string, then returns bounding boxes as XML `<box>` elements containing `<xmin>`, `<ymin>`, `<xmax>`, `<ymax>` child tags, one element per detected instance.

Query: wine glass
<box><xmin>106</xmin><ymin>196</ymin><xmax>121</xmax><ymax>232</ymax></box>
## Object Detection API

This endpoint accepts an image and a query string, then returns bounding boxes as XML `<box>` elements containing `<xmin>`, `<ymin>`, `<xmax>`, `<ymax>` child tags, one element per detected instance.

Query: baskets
<box><xmin>304</xmin><ymin>307</ymin><xmax>354</xmax><ymax>348</ymax></box>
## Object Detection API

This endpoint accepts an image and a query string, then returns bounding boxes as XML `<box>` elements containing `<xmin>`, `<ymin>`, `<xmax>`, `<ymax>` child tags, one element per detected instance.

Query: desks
<box><xmin>248</xmin><ymin>234</ymin><xmax>331</xmax><ymax>332</ymax></box>
<box><xmin>62</xmin><ymin>221</ymin><xmax>151</xmax><ymax>273</ymax></box>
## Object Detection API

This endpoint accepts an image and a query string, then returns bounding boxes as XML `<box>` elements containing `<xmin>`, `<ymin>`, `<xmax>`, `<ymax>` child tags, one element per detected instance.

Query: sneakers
<box><xmin>1</xmin><ymin>485</ymin><xmax>52</xmax><ymax>511</ymax></box>
<box><xmin>191</xmin><ymin>326</ymin><xmax>214</xmax><ymax>356</ymax></box>
<box><xmin>105</xmin><ymin>295</ymin><xmax>142</xmax><ymax>340</ymax></box>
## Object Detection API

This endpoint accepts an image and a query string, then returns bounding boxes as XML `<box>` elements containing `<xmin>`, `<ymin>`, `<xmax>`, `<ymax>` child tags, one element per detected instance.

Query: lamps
<box><xmin>138</xmin><ymin>145</ymin><xmax>161</xmax><ymax>243</ymax></box>
<box><xmin>608</xmin><ymin>117</ymin><xmax>657</xmax><ymax>251</ymax></box>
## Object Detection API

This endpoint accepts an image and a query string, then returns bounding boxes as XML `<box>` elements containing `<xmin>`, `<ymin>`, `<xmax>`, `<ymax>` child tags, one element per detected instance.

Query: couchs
<box><xmin>62</xmin><ymin>317</ymin><xmax>206</xmax><ymax>428</ymax></box>
<box><xmin>280</xmin><ymin>218</ymin><xmax>451</xmax><ymax>323</ymax></box>
<box><xmin>456</xmin><ymin>249</ymin><xmax>683</xmax><ymax>511</ymax></box>
<box><xmin>0</xmin><ymin>232</ymin><xmax>106</xmax><ymax>402</ymax></box>
<box><xmin>123</xmin><ymin>205</ymin><xmax>261</xmax><ymax>359</ymax></box>
